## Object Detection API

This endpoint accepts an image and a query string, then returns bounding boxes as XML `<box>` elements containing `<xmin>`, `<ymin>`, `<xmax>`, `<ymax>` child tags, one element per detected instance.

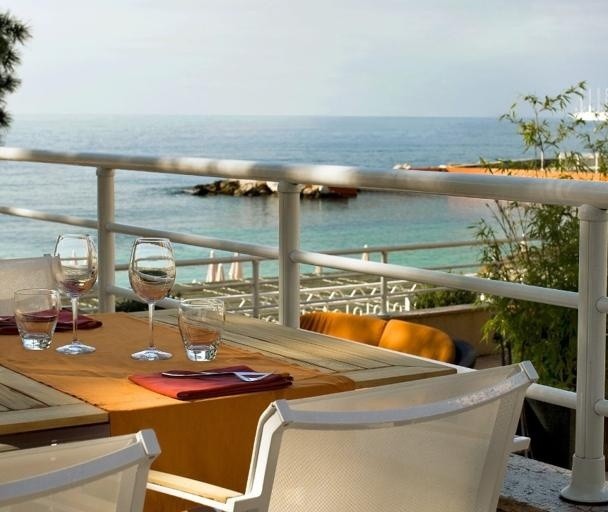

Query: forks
<box><xmin>161</xmin><ymin>372</ymin><xmax>272</xmax><ymax>382</ymax></box>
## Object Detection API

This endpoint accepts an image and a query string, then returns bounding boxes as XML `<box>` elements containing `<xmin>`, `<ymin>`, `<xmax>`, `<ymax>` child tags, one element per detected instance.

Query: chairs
<box><xmin>0</xmin><ymin>430</ymin><xmax>161</xmax><ymax>512</ymax></box>
<box><xmin>1</xmin><ymin>255</ymin><xmax>65</xmax><ymax>316</ymax></box>
<box><xmin>145</xmin><ymin>362</ymin><xmax>536</xmax><ymax>512</ymax></box>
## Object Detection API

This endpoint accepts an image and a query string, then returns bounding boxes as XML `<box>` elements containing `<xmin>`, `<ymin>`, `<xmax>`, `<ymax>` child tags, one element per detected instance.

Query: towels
<box><xmin>0</xmin><ymin>307</ymin><xmax>102</xmax><ymax>335</ymax></box>
<box><xmin>127</xmin><ymin>365</ymin><xmax>293</xmax><ymax>400</ymax></box>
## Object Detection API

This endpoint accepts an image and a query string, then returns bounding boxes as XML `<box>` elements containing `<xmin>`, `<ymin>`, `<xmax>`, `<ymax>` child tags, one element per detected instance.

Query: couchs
<box><xmin>300</xmin><ymin>312</ymin><xmax>476</xmax><ymax>369</ymax></box>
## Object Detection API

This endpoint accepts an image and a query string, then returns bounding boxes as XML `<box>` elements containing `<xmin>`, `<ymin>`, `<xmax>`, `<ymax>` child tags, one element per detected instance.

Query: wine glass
<box><xmin>51</xmin><ymin>235</ymin><xmax>98</xmax><ymax>355</ymax></box>
<box><xmin>129</xmin><ymin>238</ymin><xmax>177</xmax><ymax>361</ymax></box>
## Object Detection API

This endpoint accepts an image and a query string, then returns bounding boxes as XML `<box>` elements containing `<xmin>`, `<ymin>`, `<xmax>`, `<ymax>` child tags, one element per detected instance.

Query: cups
<box><xmin>178</xmin><ymin>298</ymin><xmax>225</xmax><ymax>361</ymax></box>
<box><xmin>15</xmin><ymin>289</ymin><xmax>59</xmax><ymax>350</ymax></box>
